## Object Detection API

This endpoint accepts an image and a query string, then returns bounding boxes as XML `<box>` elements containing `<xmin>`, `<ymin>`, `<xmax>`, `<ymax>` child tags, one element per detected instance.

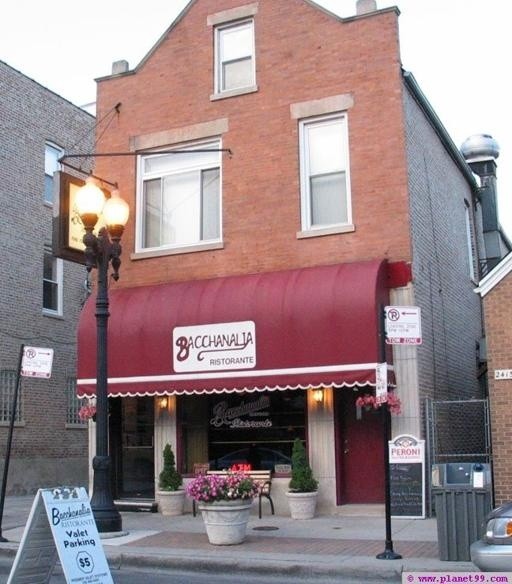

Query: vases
<box><xmin>197</xmin><ymin>499</ymin><xmax>249</xmax><ymax>545</ymax></box>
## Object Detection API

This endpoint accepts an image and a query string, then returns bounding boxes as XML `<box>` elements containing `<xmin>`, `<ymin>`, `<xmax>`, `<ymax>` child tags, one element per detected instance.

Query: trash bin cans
<box><xmin>430</xmin><ymin>463</ymin><xmax>492</xmax><ymax>561</ymax></box>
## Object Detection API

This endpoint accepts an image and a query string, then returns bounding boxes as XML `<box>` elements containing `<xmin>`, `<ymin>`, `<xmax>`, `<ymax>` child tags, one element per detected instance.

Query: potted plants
<box><xmin>284</xmin><ymin>437</ymin><xmax>319</xmax><ymax>520</ymax></box>
<box><xmin>156</xmin><ymin>443</ymin><xmax>185</xmax><ymax>516</ymax></box>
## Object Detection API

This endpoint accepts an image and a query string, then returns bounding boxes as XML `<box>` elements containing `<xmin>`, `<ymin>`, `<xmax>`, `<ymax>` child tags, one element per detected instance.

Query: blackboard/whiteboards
<box><xmin>389</xmin><ymin>463</ymin><xmax>425</xmax><ymax>519</ymax></box>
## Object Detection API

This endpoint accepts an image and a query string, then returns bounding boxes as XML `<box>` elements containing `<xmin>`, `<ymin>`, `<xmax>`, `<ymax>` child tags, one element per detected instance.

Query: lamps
<box><xmin>158</xmin><ymin>395</ymin><xmax>168</xmax><ymax>409</ymax></box>
<box><xmin>311</xmin><ymin>387</ymin><xmax>324</xmax><ymax>404</ymax></box>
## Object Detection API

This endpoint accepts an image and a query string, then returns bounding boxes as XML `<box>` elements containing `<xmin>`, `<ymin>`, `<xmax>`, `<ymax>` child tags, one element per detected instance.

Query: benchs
<box><xmin>193</xmin><ymin>470</ymin><xmax>274</xmax><ymax>518</ymax></box>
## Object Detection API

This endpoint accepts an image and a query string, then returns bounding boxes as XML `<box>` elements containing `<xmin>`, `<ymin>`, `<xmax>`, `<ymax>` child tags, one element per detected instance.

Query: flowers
<box><xmin>357</xmin><ymin>392</ymin><xmax>401</xmax><ymax>417</ymax></box>
<box><xmin>189</xmin><ymin>472</ymin><xmax>264</xmax><ymax>505</ymax></box>
<box><xmin>78</xmin><ymin>404</ymin><xmax>96</xmax><ymax>421</ymax></box>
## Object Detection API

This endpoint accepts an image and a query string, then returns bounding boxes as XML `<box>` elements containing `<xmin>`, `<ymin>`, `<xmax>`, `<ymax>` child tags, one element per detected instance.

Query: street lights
<box><xmin>73</xmin><ymin>175</ymin><xmax>130</xmax><ymax>533</ymax></box>
